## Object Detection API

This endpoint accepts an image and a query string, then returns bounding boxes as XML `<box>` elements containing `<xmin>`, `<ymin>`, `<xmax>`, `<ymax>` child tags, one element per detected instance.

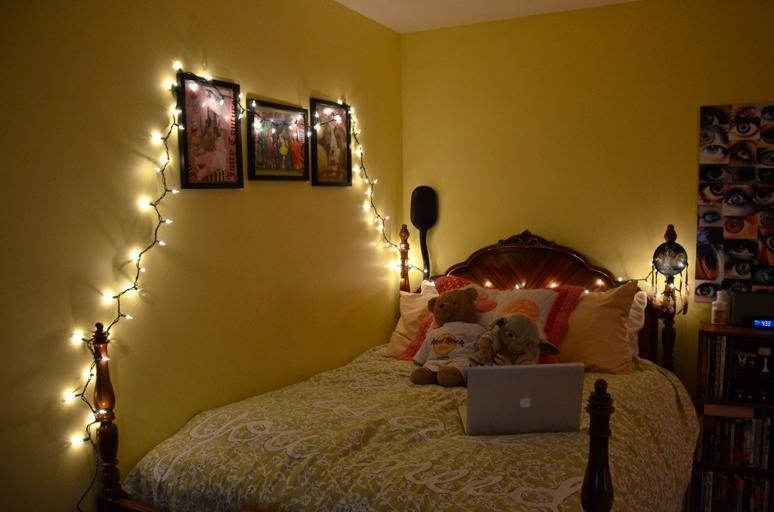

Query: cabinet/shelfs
<box><xmin>694</xmin><ymin>318</ymin><xmax>772</xmax><ymax>511</ymax></box>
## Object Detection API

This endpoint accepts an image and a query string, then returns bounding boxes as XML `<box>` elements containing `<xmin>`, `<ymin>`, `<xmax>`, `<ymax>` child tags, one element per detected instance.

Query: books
<box><xmin>687</xmin><ymin>332</ymin><xmax>773</xmax><ymax>510</ymax></box>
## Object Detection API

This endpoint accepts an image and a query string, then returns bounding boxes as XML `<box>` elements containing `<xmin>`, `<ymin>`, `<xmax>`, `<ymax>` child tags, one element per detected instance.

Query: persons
<box><xmin>253</xmin><ymin>116</ymin><xmax>303</xmax><ymax>171</ymax></box>
<box><xmin>333</xmin><ymin>126</ymin><xmax>348</xmax><ymax>182</ymax></box>
<box><xmin>316</xmin><ymin>123</ymin><xmax>335</xmax><ymax>182</ymax></box>
<box><xmin>187</xmin><ymin>99</ymin><xmax>228</xmax><ymax>182</ymax></box>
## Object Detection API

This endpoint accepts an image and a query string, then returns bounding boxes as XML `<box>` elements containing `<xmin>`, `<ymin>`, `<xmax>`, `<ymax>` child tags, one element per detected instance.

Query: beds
<box><xmin>95</xmin><ymin>223</ymin><xmax>699</xmax><ymax>512</ymax></box>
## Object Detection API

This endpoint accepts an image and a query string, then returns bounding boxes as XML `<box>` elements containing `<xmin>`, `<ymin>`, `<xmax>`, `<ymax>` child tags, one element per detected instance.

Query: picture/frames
<box><xmin>245</xmin><ymin>94</ymin><xmax>311</xmax><ymax>183</ymax></box>
<box><xmin>308</xmin><ymin>96</ymin><xmax>352</xmax><ymax>188</ymax></box>
<box><xmin>175</xmin><ymin>73</ymin><xmax>244</xmax><ymax>190</ymax></box>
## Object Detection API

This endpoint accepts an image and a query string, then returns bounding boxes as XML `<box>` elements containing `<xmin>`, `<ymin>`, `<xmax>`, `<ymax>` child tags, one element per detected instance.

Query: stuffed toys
<box><xmin>409</xmin><ymin>287</ymin><xmax>484</xmax><ymax>385</ymax></box>
<box><xmin>472</xmin><ymin>313</ymin><xmax>558</xmax><ymax>368</ymax></box>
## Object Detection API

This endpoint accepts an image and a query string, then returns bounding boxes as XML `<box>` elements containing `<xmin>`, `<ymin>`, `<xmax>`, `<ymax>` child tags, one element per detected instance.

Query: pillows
<box><xmin>382</xmin><ymin>276</ymin><xmax>647</xmax><ymax>373</ymax></box>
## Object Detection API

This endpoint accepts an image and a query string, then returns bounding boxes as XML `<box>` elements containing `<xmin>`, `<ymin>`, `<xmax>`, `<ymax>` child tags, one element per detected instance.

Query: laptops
<box><xmin>457</xmin><ymin>362</ymin><xmax>584</xmax><ymax>434</ymax></box>
